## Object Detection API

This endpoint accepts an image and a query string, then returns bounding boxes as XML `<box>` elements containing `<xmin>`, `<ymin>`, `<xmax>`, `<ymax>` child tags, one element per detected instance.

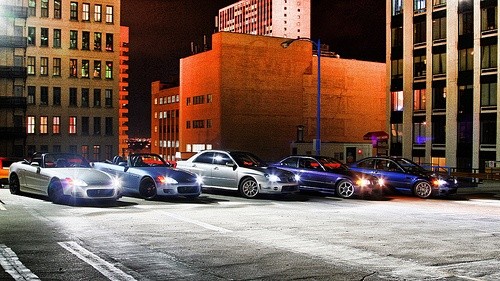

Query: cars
<box><xmin>269</xmin><ymin>154</ymin><xmax>380</xmax><ymax>196</ymax></box>
<box><xmin>173</xmin><ymin>149</ymin><xmax>301</xmax><ymax>197</ymax></box>
<box><xmin>348</xmin><ymin>156</ymin><xmax>459</xmax><ymax>197</ymax></box>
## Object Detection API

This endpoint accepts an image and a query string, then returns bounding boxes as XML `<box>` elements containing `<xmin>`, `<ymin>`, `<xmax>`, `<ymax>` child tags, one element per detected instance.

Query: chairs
<box><xmin>370</xmin><ymin>161</ymin><xmax>382</xmax><ymax>169</ymax></box>
<box><xmin>296</xmin><ymin>159</ymin><xmax>308</xmax><ymax>168</ymax></box>
<box><xmin>119</xmin><ymin>161</ymin><xmax>128</xmax><ymax>167</ymax></box>
<box><xmin>389</xmin><ymin>161</ymin><xmax>400</xmax><ymax>172</ymax></box>
<box><xmin>45</xmin><ymin>162</ymin><xmax>53</xmax><ymax>168</ymax></box>
<box><xmin>56</xmin><ymin>159</ymin><xmax>67</xmax><ymax>167</ymax></box>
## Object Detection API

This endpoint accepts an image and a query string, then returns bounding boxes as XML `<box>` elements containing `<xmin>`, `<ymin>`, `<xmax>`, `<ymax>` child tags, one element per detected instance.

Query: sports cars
<box><xmin>93</xmin><ymin>151</ymin><xmax>200</xmax><ymax>199</ymax></box>
<box><xmin>8</xmin><ymin>151</ymin><xmax>122</xmax><ymax>205</ymax></box>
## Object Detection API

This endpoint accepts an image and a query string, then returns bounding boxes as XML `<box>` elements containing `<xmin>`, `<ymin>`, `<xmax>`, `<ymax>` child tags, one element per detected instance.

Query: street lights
<box><xmin>280</xmin><ymin>36</ymin><xmax>322</xmax><ymax>155</ymax></box>
